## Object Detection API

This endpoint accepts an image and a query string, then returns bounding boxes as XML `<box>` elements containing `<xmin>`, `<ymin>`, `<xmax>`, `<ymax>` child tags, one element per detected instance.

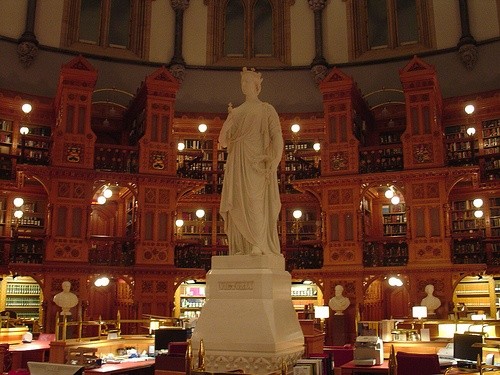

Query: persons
<box><xmin>329</xmin><ymin>284</ymin><xmax>350</xmax><ymax>315</ymax></box>
<box><xmin>218</xmin><ymin>68</ymin><xmax>283</xmax><ymax>256</ymax></box>
<box><xmin>421</xmin><ymin>285</ymin><xmax>441</xmax><ymax>314</ymax></box>
<box><xmin>52</xmin><ymin>280</ymin><xmax>78</xmax><ymax>316</ymax></box>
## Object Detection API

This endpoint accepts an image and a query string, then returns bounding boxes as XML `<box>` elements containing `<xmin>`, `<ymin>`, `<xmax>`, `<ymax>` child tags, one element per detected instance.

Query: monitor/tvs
<box><xmin>155</xmin><ymin>329</ymin><xmax>187</xmax><ymax>350</ymax></box>
<box><xmin>454</xmin><ymin>334</ymin><xmax>483</xmax><ymax>363</ymax></box>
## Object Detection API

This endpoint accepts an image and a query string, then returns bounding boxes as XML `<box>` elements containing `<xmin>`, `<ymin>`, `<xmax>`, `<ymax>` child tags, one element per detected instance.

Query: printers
<box><xmin>354</xmin><ymin>336</ymin><xmax>383</xmax><ymax>365</ymax></box>
